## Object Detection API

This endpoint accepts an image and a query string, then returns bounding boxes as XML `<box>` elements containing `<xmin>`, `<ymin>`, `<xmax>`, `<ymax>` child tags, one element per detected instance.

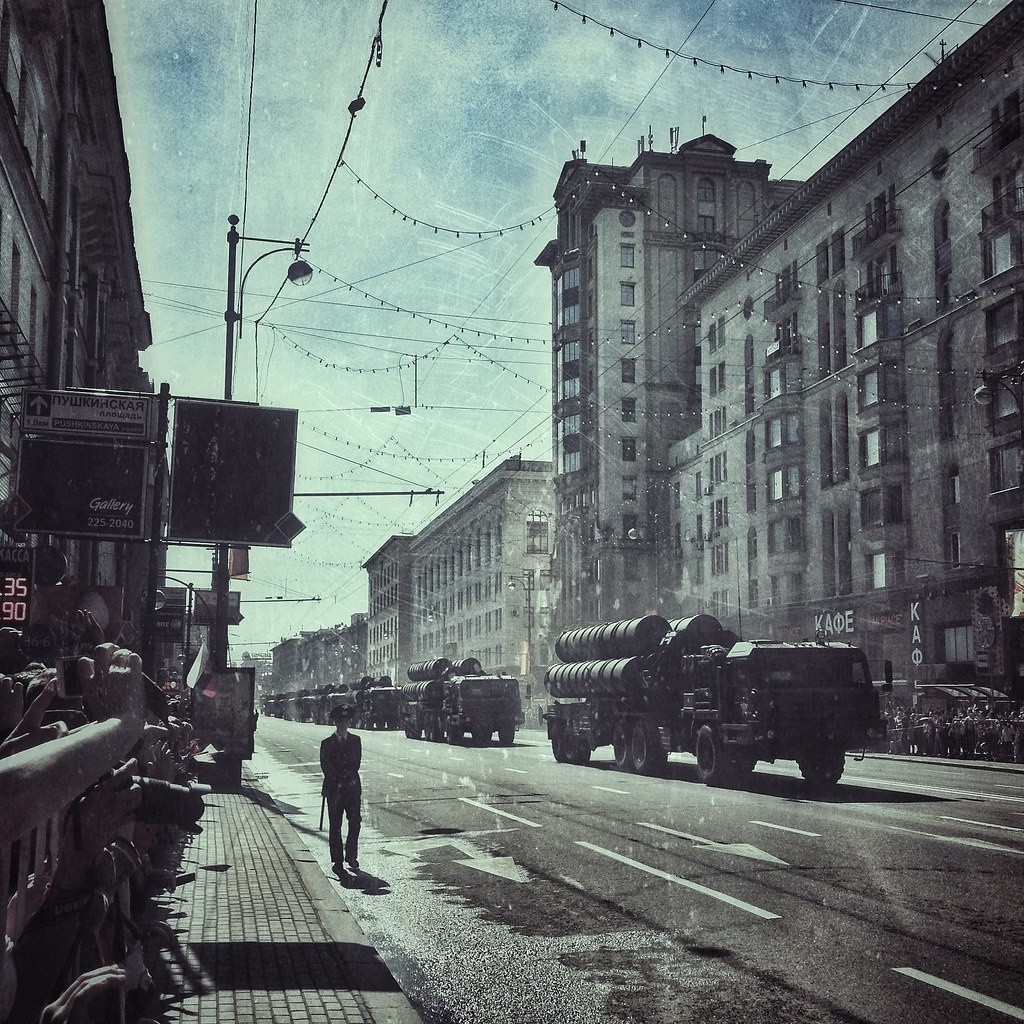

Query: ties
<box><xmin>340</xmin><ymin>736</ymin><xmax>346</xmax><ymax>743</ymax></box>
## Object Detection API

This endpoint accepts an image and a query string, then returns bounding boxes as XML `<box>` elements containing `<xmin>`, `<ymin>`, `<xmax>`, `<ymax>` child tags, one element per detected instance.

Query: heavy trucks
<box><xmin>538</xmin><ymin>614</ymin><xmax>894</xmax><ymax>790</ymax></box>
<box><xmin>258</xmin><ymin>675</ymin><xmax>402</xmax><ymax>731</ymax></box>
<box><xmin>399</xmin><ymin>657</ymin><xmax>526</xmax><ymax>747</ymax></box>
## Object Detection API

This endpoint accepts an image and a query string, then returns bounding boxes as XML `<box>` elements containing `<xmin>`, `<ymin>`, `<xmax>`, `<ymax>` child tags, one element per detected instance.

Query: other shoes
<box><xmin>345</xmin><ymin>856</ymin><xmax>359</xmax><ymax>868</ymax></box>
<box><xmin>891</xmin><ymin>750</ymin><xmax>1023</xmax><ymax>764</ymax></box>
<box><xmin>332</xmin><ymin>863</ymin><xmax>343</xmax><ymax>871</ymax></box>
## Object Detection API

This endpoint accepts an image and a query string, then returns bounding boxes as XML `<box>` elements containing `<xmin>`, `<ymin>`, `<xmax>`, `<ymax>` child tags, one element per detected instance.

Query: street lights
<box><xmin>506</xmin><ymin>574</ymin><xmax>533</xmax><ymax>700</ymax></box>
<box><xmin>629</xmin><ymin>514</ymin><xmax>661</xmax><ymax>614</ymax></box>
<box><xmin>214</xmin><ymin>213</ymin><xmax>316</xmax><ymax>669</ymax></box>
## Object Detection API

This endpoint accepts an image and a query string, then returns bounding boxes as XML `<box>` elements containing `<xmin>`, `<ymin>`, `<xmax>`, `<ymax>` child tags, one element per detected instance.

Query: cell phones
<box><xmin>56</xmin><ymin>656</ymin><xmax>93</xmax><ymax>699</ymax></box>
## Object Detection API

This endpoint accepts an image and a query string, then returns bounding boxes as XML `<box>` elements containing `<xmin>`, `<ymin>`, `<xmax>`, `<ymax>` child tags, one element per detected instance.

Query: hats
<box><xmin>330</xmin><ymin>704</ymin><xmax>354</xmax><ymax>719</ymax></box>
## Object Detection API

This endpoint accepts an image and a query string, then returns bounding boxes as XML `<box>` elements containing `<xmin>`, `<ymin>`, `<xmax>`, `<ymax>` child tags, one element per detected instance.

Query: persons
<box><xmin>881</xmin><ymin>700</ymin><xmax>1024</xmax><ymax>763</ymax></box>
<box><xmin>319</xmin><ymin>704</ymin><xmax>362</xmax><ymax>871</ymax></box>
<box><xmin>1</xmin><ymin>574</ymin><xmax>205</xmax><ymax>1024</ymax></box>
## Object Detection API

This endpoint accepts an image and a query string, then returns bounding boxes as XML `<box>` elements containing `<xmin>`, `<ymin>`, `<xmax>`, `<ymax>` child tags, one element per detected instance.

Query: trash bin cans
<box><xmin>870</xmin><ymin>719</ymin><xmax>888</xmax><ymax>753</ymax></box>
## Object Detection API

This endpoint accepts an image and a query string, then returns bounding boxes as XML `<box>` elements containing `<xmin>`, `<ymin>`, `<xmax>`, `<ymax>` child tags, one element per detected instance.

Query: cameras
<box><xmin>102</xmin><ymin>760</ymin><xmax>212</xmax><ymax>835</ymax></box>
